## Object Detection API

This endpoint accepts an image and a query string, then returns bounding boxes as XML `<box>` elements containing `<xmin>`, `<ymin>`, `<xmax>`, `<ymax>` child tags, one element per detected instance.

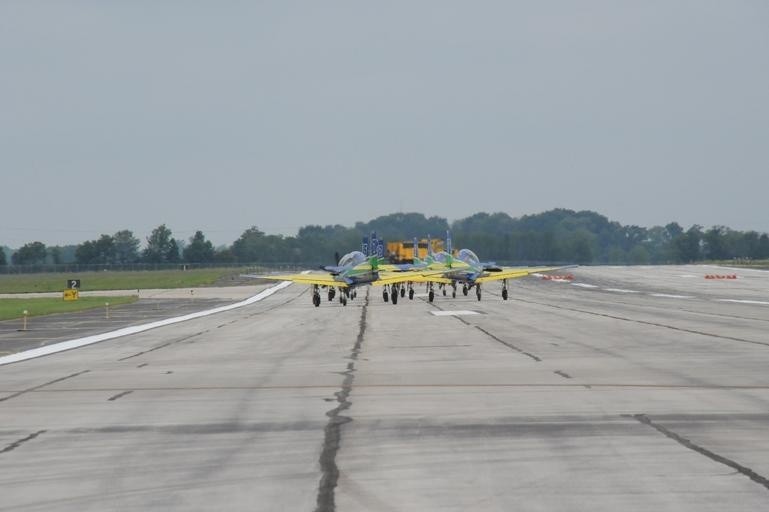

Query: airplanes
<box><xmin>237</xmin><ymin>230</ymin><xmax>580</xmax><ymax>307</ymax></box>
